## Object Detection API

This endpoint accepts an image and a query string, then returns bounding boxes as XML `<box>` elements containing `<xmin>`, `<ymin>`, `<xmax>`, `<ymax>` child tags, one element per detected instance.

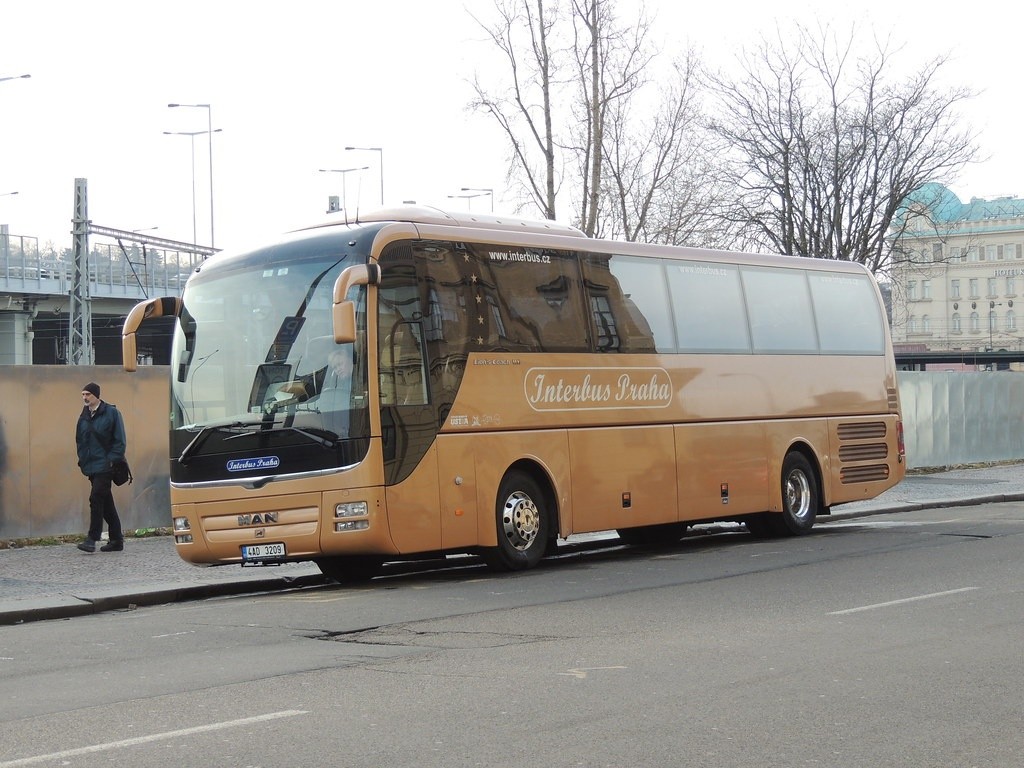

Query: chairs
<box><xmin>379</xmin><ymin>331</ymin><xmax>655</xmax><ymax>406</ymax></box>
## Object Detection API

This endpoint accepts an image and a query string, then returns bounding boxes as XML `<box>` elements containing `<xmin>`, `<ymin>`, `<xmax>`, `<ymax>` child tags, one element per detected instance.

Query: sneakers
<box><xmin>78</xmin><ymin>542</ymin><xmax>95</xmax><ymax>552</ymax></box>
<box><xmin>100</xmin><ymin>540</ymin><xmax>123</xmax><ymax>552</ymax></box>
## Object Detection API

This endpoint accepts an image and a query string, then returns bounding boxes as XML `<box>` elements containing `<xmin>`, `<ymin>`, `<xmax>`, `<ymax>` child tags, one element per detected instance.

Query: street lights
<box><xmin>461</xmin><ymin>187</ymin><xmax>493</xmax><ymax>212</ymax></box>
<box><xmin>318</xmin><ymin>166</ymin><xmax>370</xmax><ymax>208</ymax></box>
<box><xmin>447</xmin><ymin>192</ymin><xmax>491</xmax><ymax>212</ymax></box>
<box><xmin>163</xmin><ymin>128</ymin><xmax>223</xmax><ymax>244</ymax></box>
<box><xmin>167</xmin><ymin>103</ymin><xmax>215</xmax><ymax>254</ymax></box>
<box><xmin>344</xmin><ymin>146</ymin><xmax>384</xmax><ymax>206</ymax></box>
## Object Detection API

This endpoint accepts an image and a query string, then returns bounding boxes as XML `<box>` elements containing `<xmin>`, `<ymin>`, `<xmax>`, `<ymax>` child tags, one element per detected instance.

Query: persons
<box><xmin>74</xmin><ymin>382</ymin><xmax>129</xmax><ymax>553</ymax></box>
<box><xmin>327</xmin><ymin>349</ymin><xmax>360</xmax><ymax>391</ymax></box>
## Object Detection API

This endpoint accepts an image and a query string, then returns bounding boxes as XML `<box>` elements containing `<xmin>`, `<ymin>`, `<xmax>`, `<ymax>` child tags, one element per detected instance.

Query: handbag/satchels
<box><xmin>113</xmin><ymin>456</ymin><xmax>132</xmax><ymax>487</ymax></box>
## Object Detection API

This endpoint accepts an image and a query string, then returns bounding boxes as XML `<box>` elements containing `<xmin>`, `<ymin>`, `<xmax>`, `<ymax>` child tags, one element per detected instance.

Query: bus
<box><xmin>121</xmin><ymin>202</ymin><xmax>906</xmax><ymax>587</ymax></box>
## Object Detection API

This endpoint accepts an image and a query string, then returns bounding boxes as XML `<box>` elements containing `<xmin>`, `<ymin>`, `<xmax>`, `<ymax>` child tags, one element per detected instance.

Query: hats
<box><xmin>83</xmin><ymin>383</ymin><xmax>100</xmax><ymax>399</ymax></box>
